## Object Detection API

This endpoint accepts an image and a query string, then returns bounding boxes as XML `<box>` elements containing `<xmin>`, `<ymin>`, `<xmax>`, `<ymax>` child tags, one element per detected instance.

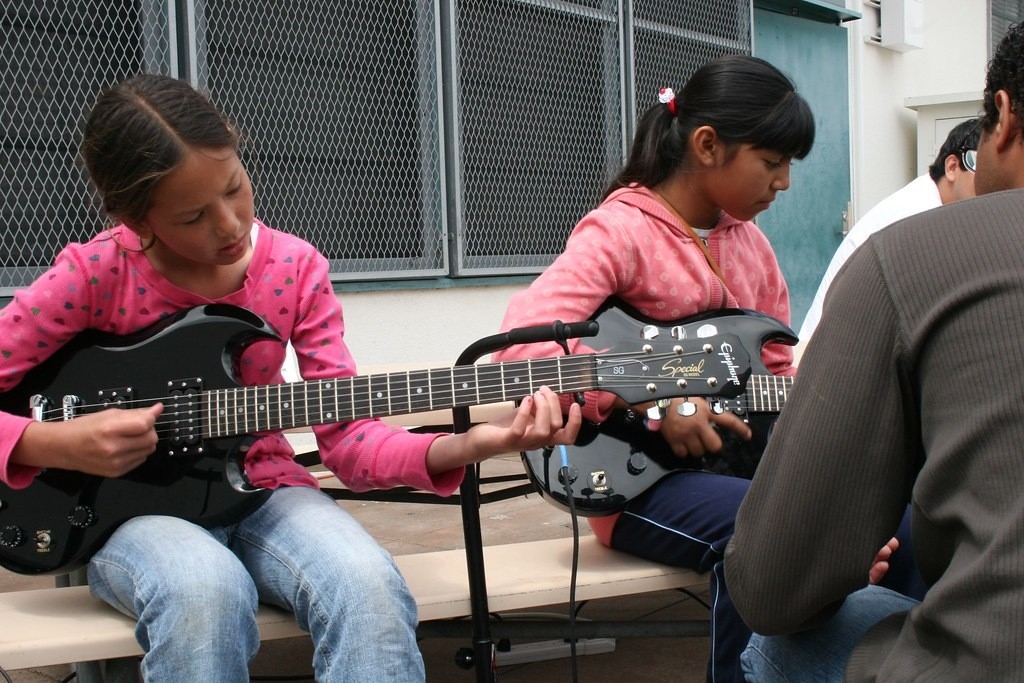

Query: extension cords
<box><xmin>495</xmin><ymin>638</ymin><xmax>615</xmax><ymax>666</ymax></box>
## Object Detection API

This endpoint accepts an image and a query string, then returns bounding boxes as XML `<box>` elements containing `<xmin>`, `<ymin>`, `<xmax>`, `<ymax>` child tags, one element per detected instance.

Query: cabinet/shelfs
<box><xmin>903</xmin><ymin>92</ymin><xmax>986</xmax><ymax>179</ymax></box>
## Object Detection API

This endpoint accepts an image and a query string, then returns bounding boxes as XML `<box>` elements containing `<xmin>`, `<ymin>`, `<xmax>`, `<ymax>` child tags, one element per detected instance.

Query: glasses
<box><xmin>958</xmin><ymin>142</ymin><xmax>980</xmax><ymax>173</ymax></box>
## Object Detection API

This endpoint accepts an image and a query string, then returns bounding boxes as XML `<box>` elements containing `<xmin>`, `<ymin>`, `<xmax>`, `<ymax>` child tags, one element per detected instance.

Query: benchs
<box><xmin>0</xmin><ymin>534</ymin><xmax>710</xmax><ymax>682</ymax></box>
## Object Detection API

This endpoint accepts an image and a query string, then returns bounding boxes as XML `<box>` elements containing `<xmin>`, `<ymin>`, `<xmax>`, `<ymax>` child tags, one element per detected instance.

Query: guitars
<box><xmin>0</xmin><ymin>299</ymin><xmax>759</xmax><ymax>574</ymax></box>
<box><xmin>510</xmin><ymin>295</ymin><xmax>799</xmax><ymax>517</ymax></box>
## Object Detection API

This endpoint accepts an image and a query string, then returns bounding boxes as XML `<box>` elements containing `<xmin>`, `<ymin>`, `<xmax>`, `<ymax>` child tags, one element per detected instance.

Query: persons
<box><xmin>723</xmin><ymin>18</ymin><xmax>1024</xmax><ymax>683</ymax></box>
<box><xmin>0</xmin><ymin>75</ymin><xmax>581</xmax><ymax>683</ymax></box>
<box><xmin>494</xmin><ymin>56</ymin><xmax>924</xmax><ymax>683</ymax></box>
<box><xmin>791</xmin><ymin>118</ymin><xmax>985</xmax><ymax>369</ymax></box>
